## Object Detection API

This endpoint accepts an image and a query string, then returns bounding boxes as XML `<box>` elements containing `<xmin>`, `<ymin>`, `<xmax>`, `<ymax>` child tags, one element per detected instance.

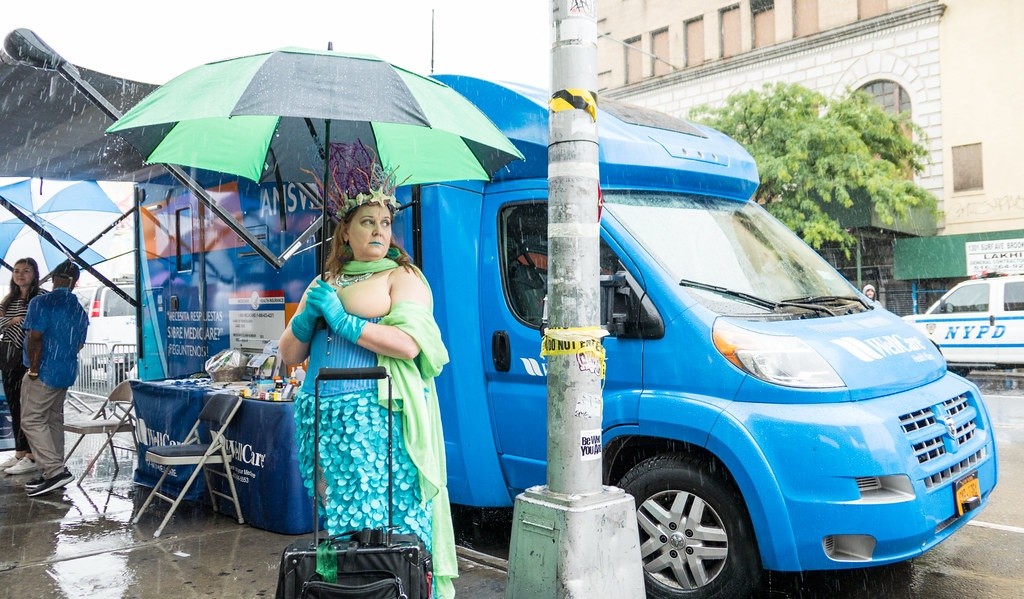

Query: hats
<box><xmin>53</xmin><ymin>261</ymin><xmax>80</xmax><ymax>279</ymax></box>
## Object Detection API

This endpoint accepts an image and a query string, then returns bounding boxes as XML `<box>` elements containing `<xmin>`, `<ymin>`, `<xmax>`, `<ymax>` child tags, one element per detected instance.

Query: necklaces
<box><xmin>336</xmin><ymin>272</ymin><xmax>374</xmax><ymax>288</ymax></box>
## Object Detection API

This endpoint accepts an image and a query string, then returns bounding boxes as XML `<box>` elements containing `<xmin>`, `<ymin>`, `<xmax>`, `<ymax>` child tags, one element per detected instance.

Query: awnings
<box><xmin>0</xmin><ymin>28</ymin><xmax>420</xmax><ymax>308</ymax></box>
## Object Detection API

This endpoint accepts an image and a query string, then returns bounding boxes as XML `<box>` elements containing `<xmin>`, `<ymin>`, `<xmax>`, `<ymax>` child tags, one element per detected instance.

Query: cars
<box><xmin>897</xmin><ymin>269</ymin><xmax>1024</xmax><ymax>380</ymax></box>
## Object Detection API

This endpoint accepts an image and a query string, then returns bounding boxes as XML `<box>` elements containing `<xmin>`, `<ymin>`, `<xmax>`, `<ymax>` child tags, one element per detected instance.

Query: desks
<box><xmin>132</xmin><ymin>372</ymin><xmax>323</xmax><ymax>535</ymax></box>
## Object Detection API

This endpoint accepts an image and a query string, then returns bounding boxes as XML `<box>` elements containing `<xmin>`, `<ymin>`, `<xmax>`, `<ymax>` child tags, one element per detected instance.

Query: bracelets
<box><xmin>26</xmin><ymin>368</ymin><xmax>38</xmax><ymax>376</ymax></box>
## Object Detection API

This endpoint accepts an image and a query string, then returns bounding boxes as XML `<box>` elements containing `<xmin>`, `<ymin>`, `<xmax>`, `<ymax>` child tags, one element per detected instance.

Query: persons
<box><xmin>280</xmin><ymin>189</ymin><xmax>460</xmax><ymax>599</ymax></box>
<box><xmin>20</xmin><ymin>261</ymin><xmax>90</xmax><ymax>497</ymax></box>
<box><xmin>863</xmin><ymin>284</ymin><xmax>881</xmax><ymax>306</ymax></box>
<box><xmin>0</xmin><ymin>258</ymin><xmax>52</xmax><ymax>474</ymax></box>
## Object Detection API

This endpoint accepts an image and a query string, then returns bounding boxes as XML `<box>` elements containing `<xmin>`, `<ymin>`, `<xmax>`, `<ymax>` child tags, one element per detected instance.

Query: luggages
<box><xmin>275</xmin><ymin>367</ymin><xmax>434</xmax><ymax>599</ymax></box>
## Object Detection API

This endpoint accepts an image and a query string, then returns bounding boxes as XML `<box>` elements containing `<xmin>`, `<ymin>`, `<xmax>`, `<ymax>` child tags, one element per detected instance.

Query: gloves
<box><xmin>291</xmin><ymin>287</ymin><xmax>338</xmax><ymax>343</ymax></box>
<box><xmin>306</xmin><ymin>279</ymin><xmax>368</xmax><ymax>344</ymax></box>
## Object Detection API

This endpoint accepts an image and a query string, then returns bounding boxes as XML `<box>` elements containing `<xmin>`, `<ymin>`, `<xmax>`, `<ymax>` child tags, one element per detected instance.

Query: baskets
<box><xmin>204</xmin><ymin>360</ymin><xmax>245</xmax><ymax>382</ymax></box>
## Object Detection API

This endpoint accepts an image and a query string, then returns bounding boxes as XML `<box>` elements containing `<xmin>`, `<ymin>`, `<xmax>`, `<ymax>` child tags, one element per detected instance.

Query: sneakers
<box><xmin>25</xmin><ymin>476</ymin><xmax>43</xmax><ymax>487</ymax></box>
<box><xmin>0</xmin><ymin>456</ymin><xmax>19</xmax><ymax>470</ymax></box>
<box><xmin>27</xmin><ymin>467</ymin><xmax>75</xmax><ymax>497</ymax></box>
<box><xmin>4</xmin><ymin>458</ymin><xmax>38</xmax><ymax>474</ymax></box>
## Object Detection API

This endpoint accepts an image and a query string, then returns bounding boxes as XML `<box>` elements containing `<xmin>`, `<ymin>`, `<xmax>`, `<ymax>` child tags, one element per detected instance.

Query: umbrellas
<box><xmin>0</xmin><ymin>178</ymin><xmax>134</xmax><ymax>281</ymax></box>
<box><xmin>108</xmin><ymin>42</ymin><xmax>526</xmax><ymax>330</ymax></box>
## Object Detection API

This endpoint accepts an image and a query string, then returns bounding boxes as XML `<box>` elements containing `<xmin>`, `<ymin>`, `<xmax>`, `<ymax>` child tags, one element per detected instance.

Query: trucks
<box><xmin>75</xmin><ymin>272</ymin><xmax>137</xmax><ymax>363</ymax></box>
<box><xmin>133</xmin><ymin>72</ymin><xmax>1003</xmax><ymax>598</ymax></box>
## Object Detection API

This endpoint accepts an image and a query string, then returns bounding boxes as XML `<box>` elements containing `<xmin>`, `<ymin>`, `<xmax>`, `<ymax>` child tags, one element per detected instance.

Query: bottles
<box><xmin>290</xmin><ymin>367</ymin><xmax>298</xmax><ymax>387</ymax></box>
<box><xmin>295</xmin><ymin>366</ymin><xmax>306</xmax><ymax>381</ymax></box>
<box><xmin>275</xmin><ymin>379</ymin><xmax>283</xmax><ymax>392</ymax></box>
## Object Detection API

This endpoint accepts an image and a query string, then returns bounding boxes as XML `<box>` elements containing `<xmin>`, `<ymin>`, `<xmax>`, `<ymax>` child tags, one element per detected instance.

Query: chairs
<box><xmin>134</xmin><ymin>394</ymin><xmax>244</xmax><ymax>538</ymax></box>
<box><xmin>63</xmin><ymin>378</ymin><xmax>136</xmax><ymax>485</ymax></box>
